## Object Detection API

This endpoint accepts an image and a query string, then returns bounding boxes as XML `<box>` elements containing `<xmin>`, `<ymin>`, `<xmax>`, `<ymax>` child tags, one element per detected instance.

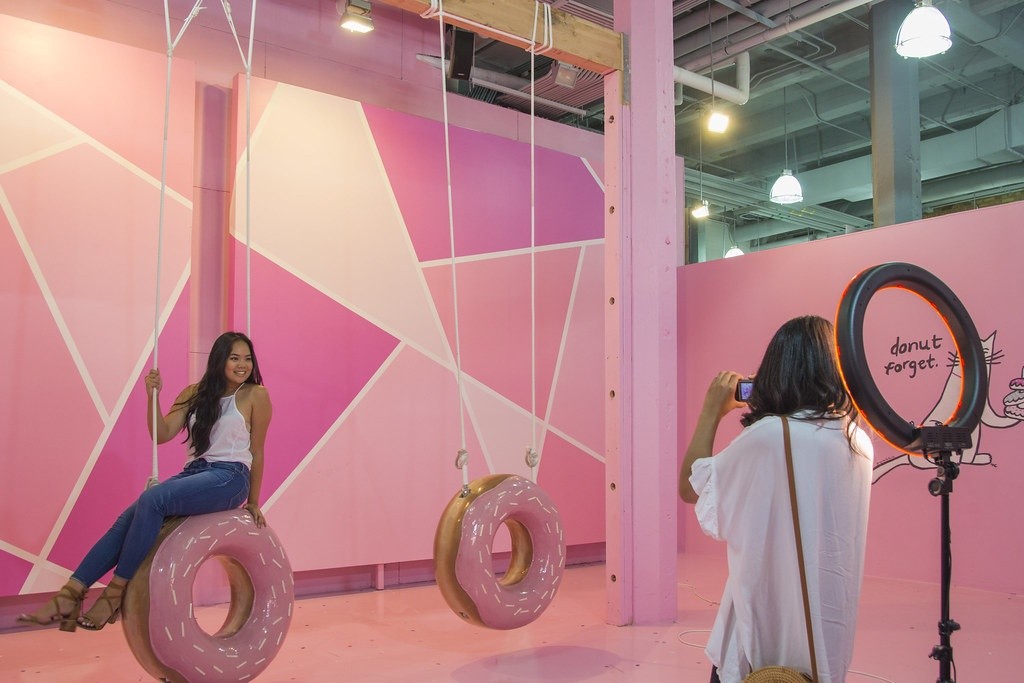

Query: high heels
<box><xmin>74</xmin><ymin>580</ymin><xmax>126</xmax><ymax>631</ymax></box>
<box><xmin>16</xmin><ymin>585</ymin><xmax>88</xmax><ymax>632</ymax></box>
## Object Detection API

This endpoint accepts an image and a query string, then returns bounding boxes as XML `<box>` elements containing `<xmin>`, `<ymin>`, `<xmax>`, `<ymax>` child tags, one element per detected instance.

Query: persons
<box><xmin>679</xmin><ymin>315</ymin><xmax>873</xmax><ymax>683</ymax></box>
<box><xmin>13</xmin><ymin>331</ymin><xmax>272</xmax><ymax>632</ymax></box>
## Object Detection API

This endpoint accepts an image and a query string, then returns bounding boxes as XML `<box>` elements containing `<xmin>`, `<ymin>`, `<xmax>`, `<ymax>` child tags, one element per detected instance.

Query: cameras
<box><xmin>736</xmin><ymin>380</ymin><xmax>755</xmax><ymax>401</ymax></box>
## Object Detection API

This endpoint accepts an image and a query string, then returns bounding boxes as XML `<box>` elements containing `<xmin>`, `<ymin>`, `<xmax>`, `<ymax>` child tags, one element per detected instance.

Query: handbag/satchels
<box><xmin>744</xmin><ymin>665</ymin><xmax>816</xmax><ymax>683</ymax></box>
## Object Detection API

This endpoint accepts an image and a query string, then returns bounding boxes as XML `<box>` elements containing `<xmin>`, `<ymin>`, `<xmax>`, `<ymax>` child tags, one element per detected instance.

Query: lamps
<box><xmin>769</xmin><ymin>87</ymin><xmax>804</xmax><ymax>204</ymax></box>
<box><xmin>894</xmin><ymin>0</ymin><xmax>953</xmax><ymax>60</ymax></box>
<box><xmin>725</xmin><ymin>210</ymin><xmax>745</xmax><ymax>258</ymax></box>
<box><xmin>707</xmin><ymin>0</ymin><xmax>729</xmax><ymax>133</ymax></box>
<box><xmin>338</xmin><ymin>0</ymin><xmax>376</xmax><ymax>35</ymax></box>
<box><xmin>550</xmin><ymin>59</ymin><xmax>580</xmax><ymax>89</ymax></box>
<box><xmin>691</xmin><ymin>109</ymin><xmax>709</xmax><ymax>219</ymax></box>
